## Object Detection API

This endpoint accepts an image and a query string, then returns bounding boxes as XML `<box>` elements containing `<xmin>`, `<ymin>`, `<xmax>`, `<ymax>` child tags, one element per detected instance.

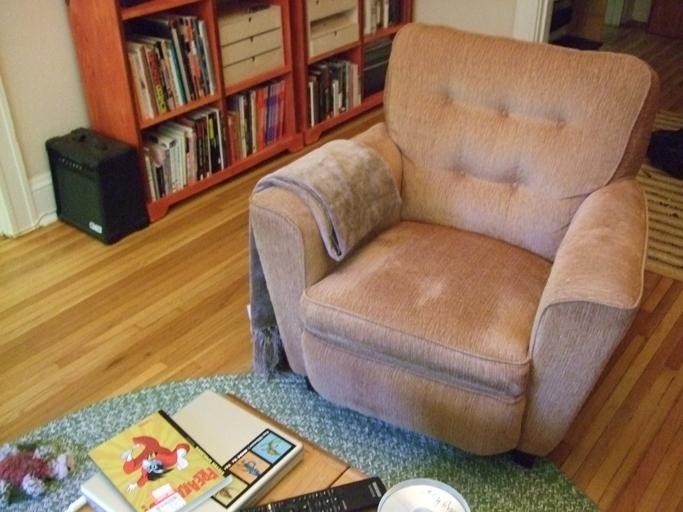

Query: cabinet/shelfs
<box><xmin>67</xmin><ymin>2</ymin><xmax>417</xmax><ymax>226</ymax></box>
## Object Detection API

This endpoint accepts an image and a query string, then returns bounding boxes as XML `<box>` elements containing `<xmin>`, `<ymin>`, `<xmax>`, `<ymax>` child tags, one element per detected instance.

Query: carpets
<box><xmin>0</xmin><ymin>366</ymin><xmax>606</xmax><ymax>512</ymax></box>
<box><xmin>631</xmin><ymin>106</ymin><xmax>683</xmax><ymax>283</ymax></box>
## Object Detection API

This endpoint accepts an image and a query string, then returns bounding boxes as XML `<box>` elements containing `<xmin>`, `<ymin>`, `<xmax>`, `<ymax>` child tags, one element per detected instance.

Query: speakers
<box><xmin>46</xmin><ymin>127</ymin><xmax>149</xmax><ymax>245</ymax></box>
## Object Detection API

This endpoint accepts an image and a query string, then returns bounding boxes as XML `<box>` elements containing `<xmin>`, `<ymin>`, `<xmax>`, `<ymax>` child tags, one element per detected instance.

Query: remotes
<box><xmin>240</xmin><ymin>476</ymin><xmax>387</xmax><ymax>512</ymax></box>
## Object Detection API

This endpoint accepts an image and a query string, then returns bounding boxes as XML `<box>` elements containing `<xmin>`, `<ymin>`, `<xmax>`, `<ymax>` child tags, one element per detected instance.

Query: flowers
<box><xmin>0</xmin><ymin>436</ymin><xmax>83</xmax><ymax>503</ymax></box>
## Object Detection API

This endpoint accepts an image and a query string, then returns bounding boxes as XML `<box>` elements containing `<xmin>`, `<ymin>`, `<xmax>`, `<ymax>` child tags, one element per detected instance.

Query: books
<box><xmin>87</xmin><ymin>409</ymin><xmax>232</xmax><ymax>512</ymax></box>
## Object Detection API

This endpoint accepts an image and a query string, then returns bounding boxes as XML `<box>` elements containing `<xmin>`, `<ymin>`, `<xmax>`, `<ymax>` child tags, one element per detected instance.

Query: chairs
<box><xmin>240</xmin><ymin>22</ymin><xmax>659</xmax><ymax>468</ymax></box>
